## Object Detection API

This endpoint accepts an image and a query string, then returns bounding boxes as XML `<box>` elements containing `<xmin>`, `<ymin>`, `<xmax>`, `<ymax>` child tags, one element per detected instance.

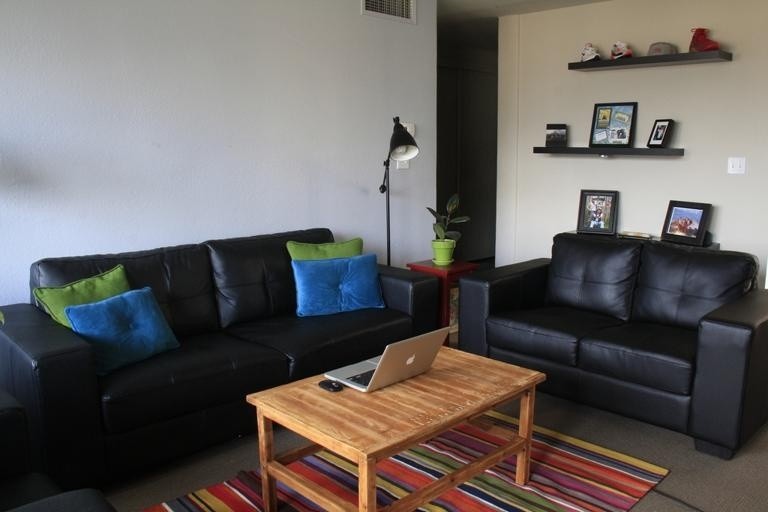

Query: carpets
<box><xmin>141</xmin><ymin>409</ymin><xmax>670</xmax><ymax>512</ymax></box>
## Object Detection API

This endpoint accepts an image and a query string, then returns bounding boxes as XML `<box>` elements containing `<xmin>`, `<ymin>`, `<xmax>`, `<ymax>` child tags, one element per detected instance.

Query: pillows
<box><xmin>290</xmin><ymin>253</ymin><xmax>386</xmax><ymax>317</ymax></box>
<box><xmin>32</xmin><ymin>264</ymin><xmax>132</xmax><ymax>331</ymax></box>
<box><xmin>64</xmin><ymin>286</ymin><xmax>181</xmax><ymax>373</ymax></box>
<box><xmin>286</xmin><ymin>238</ymin><xmax>364</xmax><ymax>260</ymax></box>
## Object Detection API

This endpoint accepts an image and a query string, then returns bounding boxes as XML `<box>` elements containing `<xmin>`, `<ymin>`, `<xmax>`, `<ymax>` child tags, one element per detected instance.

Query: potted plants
<box><xmin>425</xmin><ymin>193</ymin><xmax>471</xmax><ymax>266</ymax></box>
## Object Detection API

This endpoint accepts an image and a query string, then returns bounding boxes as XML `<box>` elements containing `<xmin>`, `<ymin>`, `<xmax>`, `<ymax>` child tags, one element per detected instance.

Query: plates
<box><xmin>431</xmin><ymin>258</ymin><xmax>455</xmax><ymax>266</ymax></box>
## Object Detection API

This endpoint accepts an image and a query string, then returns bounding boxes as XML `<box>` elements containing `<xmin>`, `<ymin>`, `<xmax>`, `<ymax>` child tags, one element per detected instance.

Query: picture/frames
<box><xmin>576</xmin><ymin>189</ymin><xmax>619</xmax><ymax>236</ymax></box>
<box><xmin>646</xmin><ymin>119</ymin><xmax>673</xmax><ymax>148</ymax></box>
<box><xmin>660</xmin><ymin>200</ymin><xmax>712</xmax><ymax>247</ymax></box>
<box><xmin>588</xmin><ymin>102</ymin><xmax>639</xmax><ymax>148</ymax></box>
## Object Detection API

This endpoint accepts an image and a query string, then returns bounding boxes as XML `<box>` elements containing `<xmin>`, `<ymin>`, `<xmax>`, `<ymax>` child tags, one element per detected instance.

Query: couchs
<box><xmin>457</xmin><ymin>232</ymin><xmax>768</xmax><ymax>461</ymax></box>
<box><xmin>0</xmin><ymin>227</ymin><xmax>445</xmax><ymax>496</ymax></box>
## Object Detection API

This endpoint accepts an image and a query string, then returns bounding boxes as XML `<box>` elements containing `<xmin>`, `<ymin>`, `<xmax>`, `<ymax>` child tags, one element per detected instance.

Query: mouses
<box><xmin>318</xmin><ymin>379</ymin><xmax>344</xmax><ymax>393</ymax></box>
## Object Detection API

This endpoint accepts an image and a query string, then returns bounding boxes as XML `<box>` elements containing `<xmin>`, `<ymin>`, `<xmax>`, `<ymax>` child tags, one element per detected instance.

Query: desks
<box><xmin>406</xmin><ymin>257</ymin><xmax>478</xmax><ymax>348</ymax></box>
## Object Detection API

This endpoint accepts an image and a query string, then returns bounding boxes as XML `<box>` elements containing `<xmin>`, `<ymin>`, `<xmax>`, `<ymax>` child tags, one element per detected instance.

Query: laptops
<box><xmin>323</xmin><ymin>326</ymin><xmax>451</xmax><ymax>393</ymax></box>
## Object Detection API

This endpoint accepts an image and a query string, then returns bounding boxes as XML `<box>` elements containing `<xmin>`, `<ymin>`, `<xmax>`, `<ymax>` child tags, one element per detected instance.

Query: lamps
<box><xmin>378</xmin><ymin>117</ymin><xmax>421</xmax><ymax>267</ymax></box>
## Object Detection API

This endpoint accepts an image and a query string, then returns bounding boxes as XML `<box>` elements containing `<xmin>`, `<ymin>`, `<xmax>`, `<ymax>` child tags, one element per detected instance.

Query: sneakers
<box><xmin>610</xmin><ymin>39</ymin><xmax>633</xmax><ymax>59</ymax></box>
<box><xmin>580</xmin><ymin>42</ymin><xmax>601</xmax><ymax>61</ymax></box>
<box><xmin>688</xmin><ymin>27</ymin><xmax>719</xmax><ymax>52</ymax></box>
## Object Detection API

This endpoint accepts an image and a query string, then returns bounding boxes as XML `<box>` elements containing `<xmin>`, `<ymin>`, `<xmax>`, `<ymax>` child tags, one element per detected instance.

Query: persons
<box><xmin>589</xmin><ymin>208</ymin><xmax>604</xmax><ymax>229</ymax></box>
